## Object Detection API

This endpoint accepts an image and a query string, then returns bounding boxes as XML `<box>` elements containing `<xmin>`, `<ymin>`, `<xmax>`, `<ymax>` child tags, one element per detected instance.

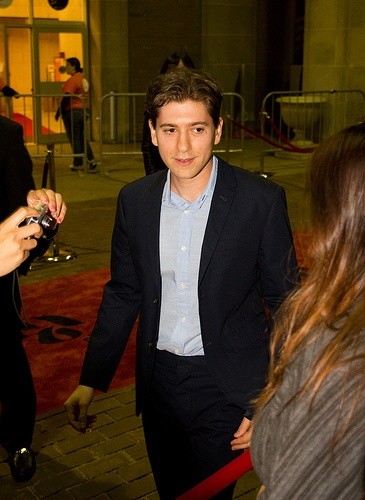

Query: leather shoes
<box><xmin>8</xmin><ymin>447</ymin><xmax>36</xmax><ymax>482</ymax></box>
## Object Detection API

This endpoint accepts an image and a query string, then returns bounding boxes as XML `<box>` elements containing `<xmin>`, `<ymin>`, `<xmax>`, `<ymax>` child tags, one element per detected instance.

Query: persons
<box><xmin>140</xmin><ymin>51</ymin><xmax>195</xmax><ymax>177</ymax></box>
<box><xmin>249</xmin><ymin>122</ymin><xmax>365</xmax><ymax>500</ymax></box>
<box><xmin>54</xmin><ymin>57</ymin><xmax>98</xmax><ymax>171</ymax></box>
<box><xmin>0</xmin><ymin>115</ymin><xmax>67</xmax><ymax>483</ymax></box>
<box><xmin>64</xmin><ymin>65</ymin><xmax>302</xmax><ymax>500</ymax></box>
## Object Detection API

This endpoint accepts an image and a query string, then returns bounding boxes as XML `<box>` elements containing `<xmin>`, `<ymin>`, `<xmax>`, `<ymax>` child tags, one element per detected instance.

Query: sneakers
<box><xmin>70</xmin><ymin>164</ymin><xmax>83</xmax><ymax>170</ymax></box>
<box><xmin>87</xmin><ymin>159</ymin><xmax>97</xmax><ymax>169</ymax></box>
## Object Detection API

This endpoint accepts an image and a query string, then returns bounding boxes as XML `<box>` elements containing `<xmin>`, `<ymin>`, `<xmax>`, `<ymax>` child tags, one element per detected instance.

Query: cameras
<box><xmin>25</xmin><ymin>202</ymin><xmax>56</xmax><ymax>240</ymax></box>
